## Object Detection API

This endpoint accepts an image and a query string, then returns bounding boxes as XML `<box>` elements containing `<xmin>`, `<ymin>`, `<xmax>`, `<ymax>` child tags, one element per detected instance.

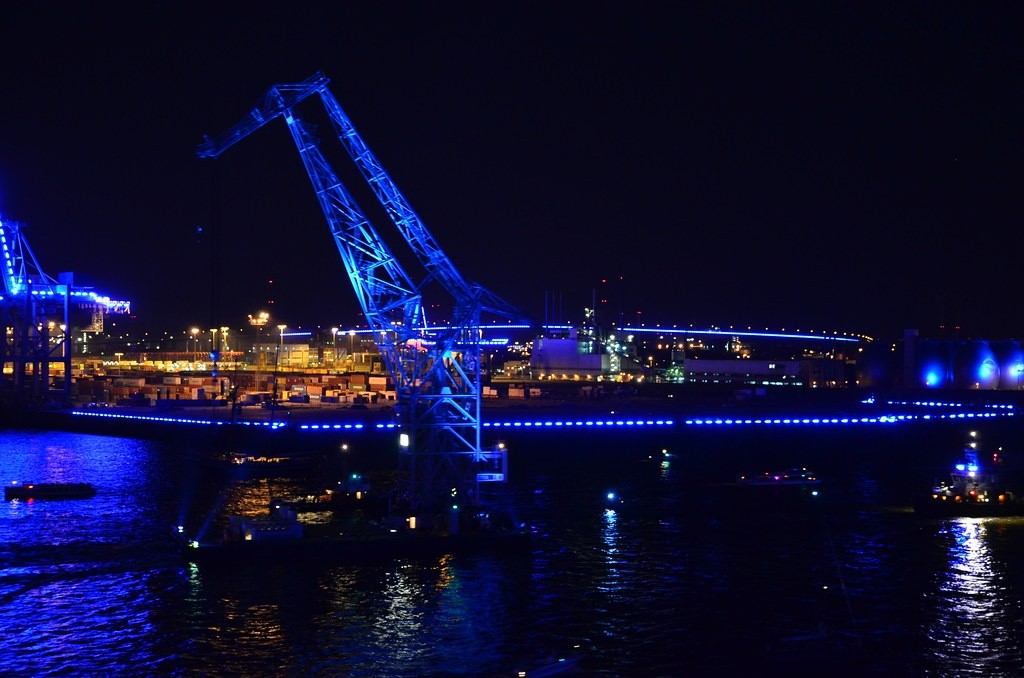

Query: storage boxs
<box><xmin>3</xmin><ymin>362</ymin><xmax>542</xmax><ymax>408</ymax></box>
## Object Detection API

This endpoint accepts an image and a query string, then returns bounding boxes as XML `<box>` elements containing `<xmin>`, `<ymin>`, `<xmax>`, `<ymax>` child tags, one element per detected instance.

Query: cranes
<box><xmin>196</xmin><ymin>69</ymin><xmax>530</xmax><ymax>486</ymax></box>
<box><xmin>0</xmin><ymin>217</ymin><xmax>132</xmax><ymax>410</ymax></box>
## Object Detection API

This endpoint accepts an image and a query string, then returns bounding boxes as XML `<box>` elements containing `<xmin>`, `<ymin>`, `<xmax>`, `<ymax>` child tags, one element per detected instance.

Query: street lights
<box><xmin>276</xmin><ymin>324</ymin><xmax>287</xmax><ymax>369</ymax></box>
<box><xmin>332</xmin><ymin>327</ymin><xmax>339</xmax><ymax>362</ymax></box>
<box><xmin>380</xmin><ymin>331</ymin><xmax>387</xmax><ymax>344</ymax></box>
<box><xmin>348</xmin><ymin>330</ymin><xmax>356</xmax><ymax>362</ymax></box>
<box><xmin>210</xmin><ymin>329</ymin><xmax>218</xmax><ymax>366</ymax></box>
<box><xmin>192</xmin><ymin>328</ymin><xmax>198</xmax><ymax>361</ymax></box>
